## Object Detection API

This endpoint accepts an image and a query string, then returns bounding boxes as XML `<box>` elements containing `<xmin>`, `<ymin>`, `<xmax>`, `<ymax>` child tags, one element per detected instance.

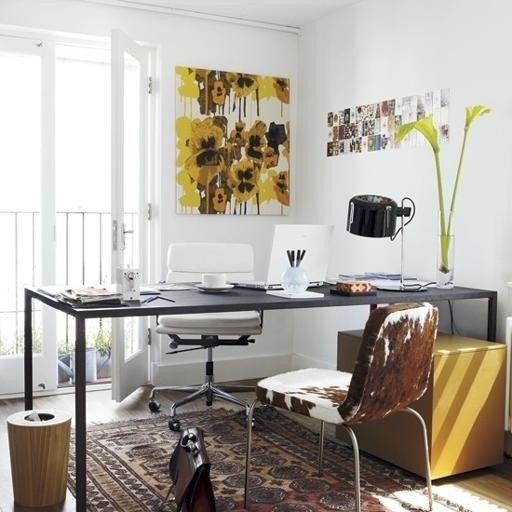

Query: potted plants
<box><xmin>392</xmin><ymin>103</ymin><xmax>492</xmax><ymax>289</ymax></box>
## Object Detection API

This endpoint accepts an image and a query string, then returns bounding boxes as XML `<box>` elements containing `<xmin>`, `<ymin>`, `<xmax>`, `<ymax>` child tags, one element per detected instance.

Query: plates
<box><xmin>198</xmin><ymin>285</ymin><xmax>235</xmax><ymax>293</ymax></box>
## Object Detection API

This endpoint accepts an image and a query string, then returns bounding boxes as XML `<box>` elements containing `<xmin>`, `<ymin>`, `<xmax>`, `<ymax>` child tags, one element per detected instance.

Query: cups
<box><xmin>202</xmin><ymin>274</ymin><xmax>226</xmax><ymax>288</ymax></box>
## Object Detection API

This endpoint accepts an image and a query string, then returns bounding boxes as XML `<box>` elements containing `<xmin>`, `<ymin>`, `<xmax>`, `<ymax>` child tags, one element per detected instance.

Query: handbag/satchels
<box><xmin>168</xmin><ymin>428</ymin><xmax>216</xmax><ymax>512</ymax></box>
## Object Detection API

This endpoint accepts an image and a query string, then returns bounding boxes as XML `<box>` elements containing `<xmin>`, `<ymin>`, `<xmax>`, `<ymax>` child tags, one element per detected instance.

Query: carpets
<box><xmin>67</xmin><ymin>404</ymin><xmax>472</xmax><ymax>512</ymax></box>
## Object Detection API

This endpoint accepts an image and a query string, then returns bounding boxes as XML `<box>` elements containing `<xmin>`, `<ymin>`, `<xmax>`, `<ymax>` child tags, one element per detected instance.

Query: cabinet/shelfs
<box><xmin>336</xmin><ymin>327</ymin><xmax>506</xmax><ymax>482</ymax></box>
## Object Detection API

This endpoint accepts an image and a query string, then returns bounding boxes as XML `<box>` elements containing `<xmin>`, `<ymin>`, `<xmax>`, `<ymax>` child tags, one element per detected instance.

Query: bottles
<box><xmin>280</xmin><ymin>268</ymin><xmax>309</xmax><ymax>294</ymax></box>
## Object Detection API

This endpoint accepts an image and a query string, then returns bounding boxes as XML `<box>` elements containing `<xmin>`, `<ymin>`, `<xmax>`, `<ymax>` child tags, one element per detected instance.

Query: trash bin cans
<box><xmin>5</xmin><ymin>409</ymin><xmax>71</xmax><ymax>507</ymax></box>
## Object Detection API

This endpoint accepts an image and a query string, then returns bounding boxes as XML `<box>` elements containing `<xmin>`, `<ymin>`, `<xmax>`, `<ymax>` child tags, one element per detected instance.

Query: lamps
<box><xmin>346</xmin><ymin>194</ymin><xmax>429</xmax><ymax>292</ymax></box>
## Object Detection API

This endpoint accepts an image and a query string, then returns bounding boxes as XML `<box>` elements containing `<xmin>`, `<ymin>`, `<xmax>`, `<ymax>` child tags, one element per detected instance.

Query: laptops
<box><xmin>230</xmin><ymin>224</ymin><xmax>331</xmax><ymax>290</ymax></box>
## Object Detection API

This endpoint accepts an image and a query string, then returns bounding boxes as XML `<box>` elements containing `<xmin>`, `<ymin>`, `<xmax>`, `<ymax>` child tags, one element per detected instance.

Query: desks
<box><xmin>23</xmin><ymin>277</ymin><xmax>497</xmax><ymax>512</ymax></box>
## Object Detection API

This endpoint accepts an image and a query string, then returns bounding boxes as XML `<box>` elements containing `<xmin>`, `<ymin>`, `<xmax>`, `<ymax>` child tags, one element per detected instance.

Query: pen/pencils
<box><xmin>287</xmin><ymin>252</ymin><xmax>305</xmax><ymax>265</ymax></box>
<box><xmin>141</xmin><ymin>295</ymin><xmax>175</xmax><ymax>309</ymax></box>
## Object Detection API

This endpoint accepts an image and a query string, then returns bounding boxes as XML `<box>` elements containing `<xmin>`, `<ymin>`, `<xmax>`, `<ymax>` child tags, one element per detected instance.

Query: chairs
<box><xmin>148</xmin><ymin>241</ymin><xmax>264</xmax><ymax>431</ymax></box>
<box><xmin>244</xmin><ymin>300</ymin><xmax>439</xmax><ymax>511</ymax></box>
